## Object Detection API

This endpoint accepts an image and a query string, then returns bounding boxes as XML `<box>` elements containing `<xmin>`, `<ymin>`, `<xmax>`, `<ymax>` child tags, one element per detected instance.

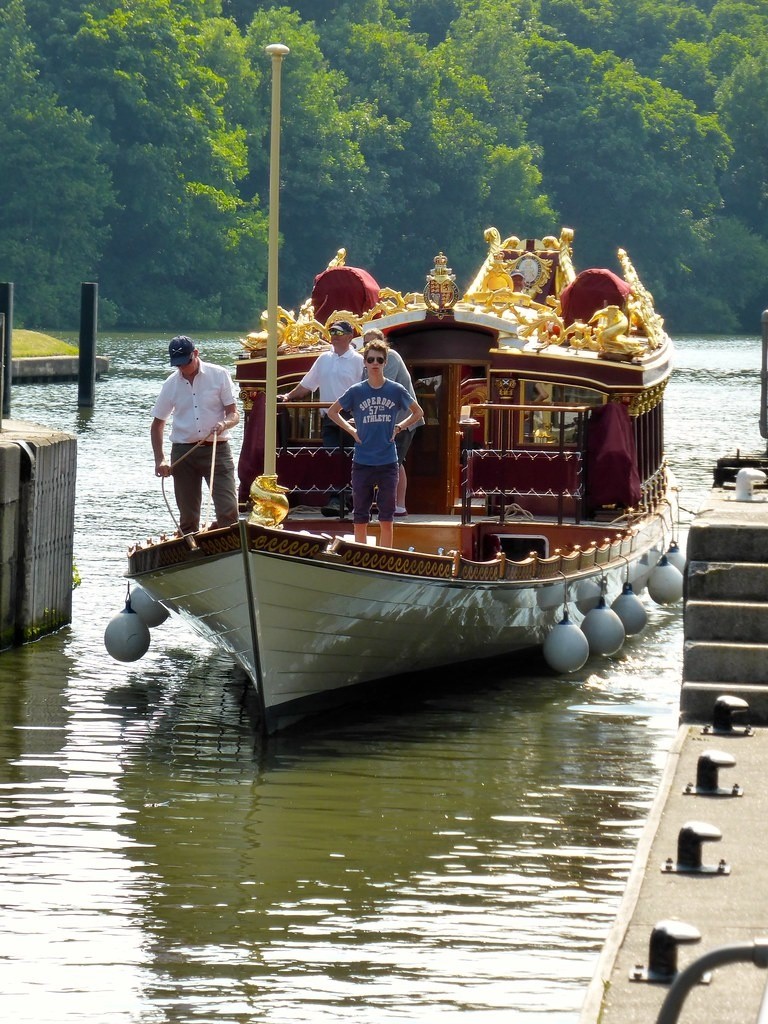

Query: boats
<box><xmin>105</xmin><ymin>225</ymin><xmax>689</xmax><ymax>738</ymax></box>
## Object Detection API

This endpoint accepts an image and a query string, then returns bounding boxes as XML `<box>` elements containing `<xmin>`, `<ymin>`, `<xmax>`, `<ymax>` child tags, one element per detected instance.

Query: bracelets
<box><xmin>395</xmin><ymin>423</ymin><xmax>402</xmax><ymax>432</ymax></box>
<box><xmin>223</xmin><ymin>420</ymin><xmax>228</xmax><ymax>429</ymax></box>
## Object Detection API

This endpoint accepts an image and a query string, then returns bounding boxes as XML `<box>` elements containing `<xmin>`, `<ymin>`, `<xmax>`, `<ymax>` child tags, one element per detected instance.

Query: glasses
<box><xmin>367</xmin><ymin>357</ymin><xmax>384</xmax><ymax>364</ymax></box>
<box><xmin>330</xmin><ymin>330</ymin><xmax>351</xmax><ymax>335</ymax></box>
<box><xmin>364</xmin><ymin>342</ymin><xmax>368</xmax><ymax>347</ymax></box>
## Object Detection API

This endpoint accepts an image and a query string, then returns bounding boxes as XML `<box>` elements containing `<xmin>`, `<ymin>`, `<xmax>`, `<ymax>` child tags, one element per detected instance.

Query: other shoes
<box><xmin>394</xmin><ymin>507</ymin><xmax>407</xmax><ymax>516</ymax></box>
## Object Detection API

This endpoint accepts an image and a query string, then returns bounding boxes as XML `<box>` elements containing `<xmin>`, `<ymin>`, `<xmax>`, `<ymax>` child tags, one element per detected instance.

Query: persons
<box><xmin>363</xmin><ymin>328</ymin><xmax>427</xmax><ymax>516</ymax></box>
<box><xmin>150</xmin><ymin>335</ymin><xmax>240</xmax><ymax>538</ymax></box>
<box><xmin>327</xmin><ymin>340</ymin><xmax>424</xmax><ymax>554</ymax></box>
<box><xmin>277</xmin><ymin>322</ymin><xmax>365</xmax><ymax>452</ymax></box>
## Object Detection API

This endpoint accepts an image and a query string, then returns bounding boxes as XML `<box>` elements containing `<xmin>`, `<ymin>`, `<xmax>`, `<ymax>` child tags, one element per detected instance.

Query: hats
<box><xmin>328</xmin><ymin>322</ymin><xmax>352</xmax><ymax>334</ymax></box>
<box><xmin>169</xmin><ymin>335</ymin><xmax>194</xmax><ymax>366</ymax></box>
